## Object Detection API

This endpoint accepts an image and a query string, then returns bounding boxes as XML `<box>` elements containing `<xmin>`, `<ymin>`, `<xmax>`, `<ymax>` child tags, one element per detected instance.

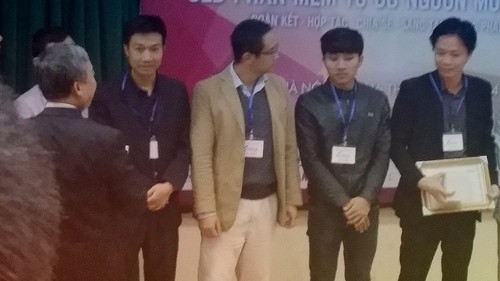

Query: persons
<box><xmin>14</xmin><ymin>26</ymin><xmax>94</xmax><ymax>123</ymax></box>
<box><xmin>191</xmin><ymin>17</ymin><xmax>303</xmax><ymax>281</ymax></box>
<box><xmin>89</xmin><ymin>14</ymin><xmax>192</xmax><ymax>281</ymax></box>
<box><xmin>389</xmin><ymin>16</ymin><xmax>499</xmax><ymax>281</ymax></box>
<box><xmin>24</xmin><ymin>40</ymin><xmax>148</xmax><ymax>281</ymax></box>
<box><xmin>294</xmin><ymin>27</ymin><xmax>393</xmax><ymax>281</ymax></box>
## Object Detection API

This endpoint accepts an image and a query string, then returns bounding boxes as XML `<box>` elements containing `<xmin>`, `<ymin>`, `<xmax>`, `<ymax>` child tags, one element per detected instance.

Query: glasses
<box><xmin>251</xmin><ymin>45</ymin><xmax>279</xmax><ymax>55</ymax></box>
